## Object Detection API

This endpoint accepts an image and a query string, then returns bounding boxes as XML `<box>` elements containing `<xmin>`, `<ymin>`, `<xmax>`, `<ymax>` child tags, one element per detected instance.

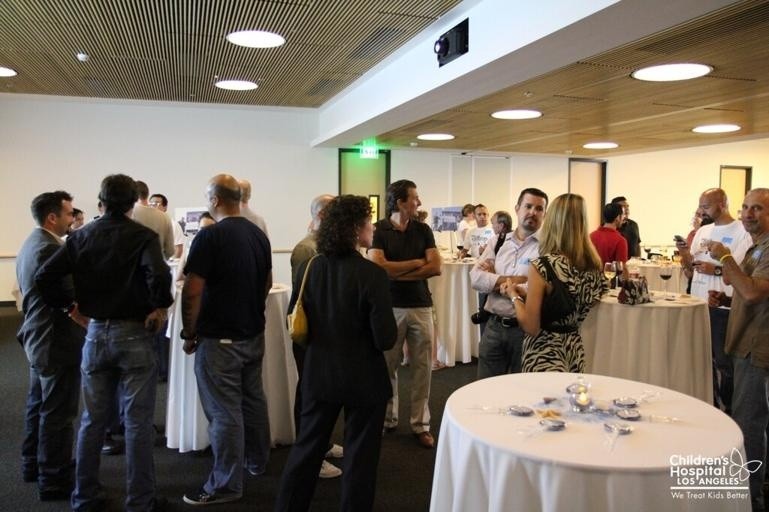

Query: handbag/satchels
<box><xmin>616</xmin><ymin>276</ymin><xmax>651</xmax><ymax>305</ymax></box>
<box><xmin>286</xmin><ymin>299</ymin><xmax>309</xmax><ymax>350</ymax></box>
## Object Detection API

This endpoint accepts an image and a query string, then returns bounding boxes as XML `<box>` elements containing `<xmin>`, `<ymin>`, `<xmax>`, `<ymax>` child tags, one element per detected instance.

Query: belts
<box><xmin>87</xmin><ymin>318</ymin><xmax>119</xmax><ymax>323</ymax></box>
<box><xmin>490</xmin><ymin>312</ymin><xmax>519</xmax><ymax>329</ymax></box>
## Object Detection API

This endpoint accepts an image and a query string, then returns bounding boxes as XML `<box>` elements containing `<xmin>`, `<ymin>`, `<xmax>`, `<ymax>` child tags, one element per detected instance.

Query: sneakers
<box><xmin>326</xmin><ymin>442</ymin><xmax>346</xmax><ymax>458</ymax></box>
<box><xmin>318</xmin><ymin>460</ymin><xmax>344</xmax><ymax>479</ymax></box>
<box><xmin>182</xmin><ymin>485</ymin><xmax>243</xmax><ymax>505</ymax></box>
<box><xmin>416</xmin><ymin>430</ymin><xmax>435</xmax><ymax>448</ymax></box>
<box><xmin>101</xmin><ymin>431</ymin><xmax>121</xmax><ymax>456</ymax></box>
<box><xmin>247</xmin><ymin>465</ymin><xmax>267</xmax><ymax>477</ymax></box>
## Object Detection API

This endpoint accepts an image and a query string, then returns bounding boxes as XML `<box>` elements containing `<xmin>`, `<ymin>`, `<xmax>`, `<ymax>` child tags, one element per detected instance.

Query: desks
<box><xmin>164</xmin><ymin>250</ymin><xmax>752</xmax><ymax>512</ymax></box>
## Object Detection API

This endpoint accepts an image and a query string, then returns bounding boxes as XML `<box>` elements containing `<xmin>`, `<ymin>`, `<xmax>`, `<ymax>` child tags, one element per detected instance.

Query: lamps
<box><xmin>434</xmin><ymin>40</ymin><xmax>447</xmax><ymax>54</ymax></box>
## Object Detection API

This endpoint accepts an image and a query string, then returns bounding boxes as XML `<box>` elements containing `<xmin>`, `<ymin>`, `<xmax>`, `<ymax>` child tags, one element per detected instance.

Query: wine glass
<box><xmin>614</xmin><ymin>260</ymin><xmax>624</xmax><ymax>292</ymax></box>
<box><xmin>603</xmin><ymin>261</ymin><xmax>617</xmax><ymax>296</ymax></box>
<box><xmin>457</xmin><ymin>239</ymin><xmax>465</xmax><ymax>262</ymax></box>
<box><xmin>644</xmin><ymin>241</ymin><xmax>652</xmax><ymax>262</ymax></box>
<box><xmin>659</xmin><ymin>263</ymin><xmax>673</xmax><ymax>298</ymax></box>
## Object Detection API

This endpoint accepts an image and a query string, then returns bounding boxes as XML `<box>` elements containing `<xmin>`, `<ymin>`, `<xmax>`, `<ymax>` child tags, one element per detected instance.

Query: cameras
<box><xmin>471</xmin><ymin>307</ymin><xmax>490</xmax><ymax>324</ymax></box>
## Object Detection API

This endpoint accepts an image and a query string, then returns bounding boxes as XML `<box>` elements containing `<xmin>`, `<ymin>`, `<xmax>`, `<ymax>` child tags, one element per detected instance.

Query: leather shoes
<box><xmin>21</xmin><ymin>461</ymin><xmax>104</xmax><ymax>512</ymax></box>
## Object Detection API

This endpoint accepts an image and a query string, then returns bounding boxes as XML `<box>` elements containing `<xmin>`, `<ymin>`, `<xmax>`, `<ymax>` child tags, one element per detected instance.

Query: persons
<box><xmin>366</xmin><ymin>181</ymin><xmax>441</xmax><ymax>449</ymax></box>
<box><xmin>275</xmin><ymin>196</ymin><xmax>398</xmax><ymax>512</ymax></box>
<box><xmin>469</xmin><ymin>189</ymin><xmax>549</xmax><ymax>380</ymax></box>
<box><xmin>499</xmin><ymin>194</ymin><xmax>609</xmax><ymax>373</ymax></box>
<box><xmin>599</xmin><ymin>197</ymin><xmax>641</xmax><ymax>260</ymax></box>
<box><xmin>589</xmin><ymin>204</ymin><xmax>629</xmax><ymax>289</ymax></box>
<box><xmin>16</xmin><ymin>174</ymin><xmax>272</xmax><ymax>512</ymax></box>
<box><xmin>456</xmin><ymin>204</ymin><xmax>515</xmax><ymax>338</ymax></box>
<box><xmin>286</xmin><ymin>196</ymin><xmax>344</xmax><ymax>478</ymax></box>
<box><xmin>674</xmin><ymin>188</ymin><xmax>769</xmax><ymax>512</ymax></box>
<box><xmin>400</xmin><ymin>211</ymin><xmax>446</xmax><ymax>371</ymax></box>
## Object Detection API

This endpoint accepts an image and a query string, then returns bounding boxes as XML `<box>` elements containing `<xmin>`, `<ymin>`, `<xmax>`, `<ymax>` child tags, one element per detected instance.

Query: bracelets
<box><xmin>511</xmin><ymin>295</ymin><xmax>524</xmax><ymax>303</ymax></box>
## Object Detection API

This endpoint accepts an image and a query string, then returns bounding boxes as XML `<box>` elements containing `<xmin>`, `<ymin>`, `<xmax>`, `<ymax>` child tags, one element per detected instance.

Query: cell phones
<box><xmin>674</xmin><ymin>235</ymin><xmax>686</xmax><ymax>245</ymax></box>
